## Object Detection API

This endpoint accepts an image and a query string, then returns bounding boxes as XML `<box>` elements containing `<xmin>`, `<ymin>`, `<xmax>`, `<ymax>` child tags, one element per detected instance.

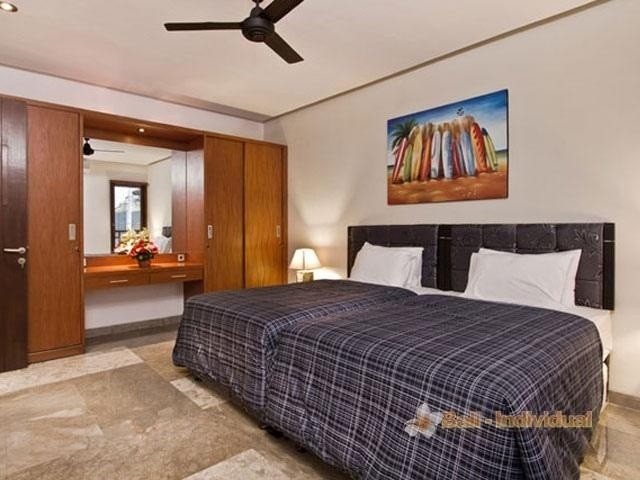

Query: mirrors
<box><xmin>83</xmin><ymin>134</ymin><xmax>175</xmax><ymax>257</ymax></box>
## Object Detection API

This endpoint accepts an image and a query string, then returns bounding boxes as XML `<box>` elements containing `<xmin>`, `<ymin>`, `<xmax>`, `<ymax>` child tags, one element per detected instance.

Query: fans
<box><xmin>83</xmin><ymin>137</ymin><xmax>127</xmax><ymax>161</ymax></box>
<box><xmin>161</xmin><ymin>0</ymin><xmax>311</xmax><ymax>66</ymax></box>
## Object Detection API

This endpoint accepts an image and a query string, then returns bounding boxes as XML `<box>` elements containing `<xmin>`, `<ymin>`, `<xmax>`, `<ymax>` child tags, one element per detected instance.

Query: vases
<box><xmin>137</xmin><ymin>258</ymin><xmax>151</xmax><ymax>267</ymax></box>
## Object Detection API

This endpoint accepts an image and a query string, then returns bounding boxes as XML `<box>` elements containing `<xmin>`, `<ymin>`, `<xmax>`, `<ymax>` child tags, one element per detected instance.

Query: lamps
<box><xmin>288</xmin><ymin>248</ymin><xmax>323</xmax><ymax>284</ymax></box>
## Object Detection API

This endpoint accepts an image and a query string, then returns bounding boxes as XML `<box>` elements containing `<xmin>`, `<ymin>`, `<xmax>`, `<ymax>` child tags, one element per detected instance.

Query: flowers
<box><xmin>119</xmin><ymin>224</ymin><xmax>148</xmax><ymax>249</ymax></box>
<box><xmin>127</xmin><ymin>238</ymin><xmax>159</xmax><ymax>261</ymax></box>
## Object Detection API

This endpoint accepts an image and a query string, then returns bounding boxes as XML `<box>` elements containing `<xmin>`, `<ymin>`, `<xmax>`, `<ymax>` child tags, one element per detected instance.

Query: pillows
<box><xmin>465</xmin><ymin>250</ymin><xmax>574</xmax><ymax>311</ymax></box>
<box><xmin>478</xmin><ymin>245</ymin><xmax>583</xmax><ymax>313</ymax></box>
<box><xmin>155</xmin><ymin>233</ymin><xmax>170</xmax><ymax>253</ymax></box>
<box><xmin>350</xmin><ymin>244</ymin><xmax>416</xmax><ymax>290</ymax></box>
<box><xmin>364</xmin><ymin>240</ymin><xmax>425</xmax><ymax>290</ymax></box>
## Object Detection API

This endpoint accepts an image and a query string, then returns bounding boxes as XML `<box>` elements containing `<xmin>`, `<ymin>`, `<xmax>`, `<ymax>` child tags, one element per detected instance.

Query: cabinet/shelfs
<box><xmin>84</xmin><ymin>264</ymin><xmax>203</xmax><ymax>288</ymax></box>
<box><xmin>185</xmin><ymin>136</ymin><xmax>243</xmax><ymax>303</ymax></box>
<box><xmin>242</xmin><ymin>143</ymin><xmax>285</xmax><ymax>289</ymax></box>
<box><xmin>27</xmin><ymin>102</ymin><xmax>84</xmax><ymax>354</ymax></box>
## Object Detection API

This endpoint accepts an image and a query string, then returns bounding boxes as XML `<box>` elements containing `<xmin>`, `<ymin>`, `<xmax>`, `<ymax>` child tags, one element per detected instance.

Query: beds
<box><xmin>171</xmin><ymin>223</ymin><xmax>447</xmax><ymax>439</ymax></box>
<box><xmin>263</xmin><ymin>221</ymin><xmax>617</xmax><ymax>480</ymax></box>
<box><xmin>84</xmin><ymin>224</ymin><xmax>172</xmax><ymax>256</ymax></box>
